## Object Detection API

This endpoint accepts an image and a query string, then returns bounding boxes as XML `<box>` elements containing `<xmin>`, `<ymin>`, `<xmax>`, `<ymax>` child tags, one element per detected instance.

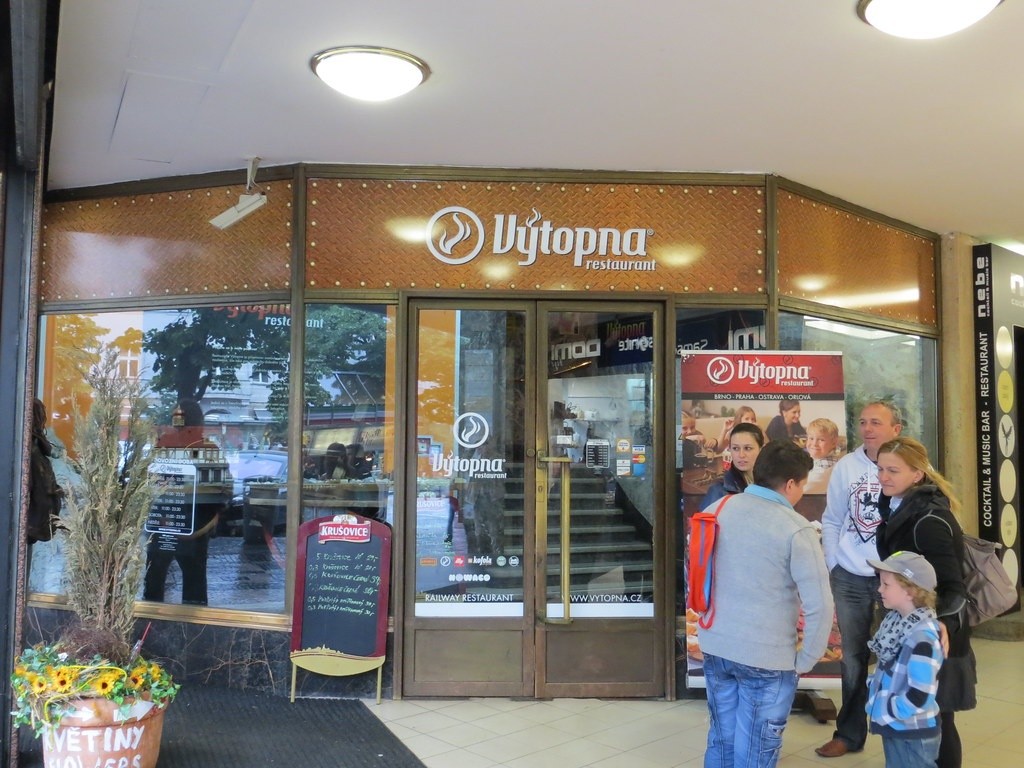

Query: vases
<box><xmin>40</xmin><ymin>689</ymin><xmax>169</xmax><ymax>768</ymax></box>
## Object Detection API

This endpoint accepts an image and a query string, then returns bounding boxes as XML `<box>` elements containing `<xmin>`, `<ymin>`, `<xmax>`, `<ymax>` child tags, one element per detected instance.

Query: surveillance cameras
<box><xmin>208</xmin><ymin>193</ymin><xmax>267</xmax><ymax>229</ymax></box>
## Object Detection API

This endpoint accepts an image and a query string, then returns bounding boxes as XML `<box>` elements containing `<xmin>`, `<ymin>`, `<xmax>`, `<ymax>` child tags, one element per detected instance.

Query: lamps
<box><xmin>310</xmin><ymin>47</ymin><xmax>431</xmax><ymax>101</ymax></box>
<box><xmin>857</xmin><ymin>0</ymin><xmax>1005</xmax><ymax>46</ymax></box>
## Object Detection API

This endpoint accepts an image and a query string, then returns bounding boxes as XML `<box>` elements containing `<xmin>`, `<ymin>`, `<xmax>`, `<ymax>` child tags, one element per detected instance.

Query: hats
<box><xmin>866</xmin><ymin>552</ymin><xmax>937</xmax><ymax>593</ymax></box>
<box><xmin>171</xmin><ymin>400</ymin><xmax>204</xmax><ymax>426</ymax></box>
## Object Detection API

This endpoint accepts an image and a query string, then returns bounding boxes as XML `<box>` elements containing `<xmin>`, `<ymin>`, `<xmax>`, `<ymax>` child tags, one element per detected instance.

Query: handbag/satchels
<box><xmin>959</xmin><ymin>530</ymin><xmax>1018</xmax><ymax>625</ymax></box>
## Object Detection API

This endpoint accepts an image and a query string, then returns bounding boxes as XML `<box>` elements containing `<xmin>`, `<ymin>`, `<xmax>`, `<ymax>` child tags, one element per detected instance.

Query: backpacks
<box><xmin>29</xmin><ymin>446</ymin><xmax>65</xmax><ymax>542</ymax></box>
<box><xmin>687</xmin><ymin>495</ymin><xmax>729</xmax><ymax>629</ymax></box>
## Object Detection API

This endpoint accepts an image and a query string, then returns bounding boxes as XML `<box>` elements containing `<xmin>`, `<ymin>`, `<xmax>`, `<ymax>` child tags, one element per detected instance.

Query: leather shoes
<box><xmin>815</xmin><ymin>738</ymin><xmax>850</xmax><ymax>757</ymax></box>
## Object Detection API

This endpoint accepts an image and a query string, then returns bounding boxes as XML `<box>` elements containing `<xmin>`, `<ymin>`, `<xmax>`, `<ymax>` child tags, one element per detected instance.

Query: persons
<box><xmin>814</xmin><ymin>401</ymin><xmax>904</xmax><ymax>759</ymax></box>
<box><xmin>700</xmin><ymin>423</ymin><xmax>766</xmax><ymax>512</ymax></box>
<box><xmin>874</xmin><ymin>437</ymin><xmax>977</xmax><ymax>768</ymax></box>
<box><xmin>865</xmin><ymin>550</ymin><xmax>943</xmax><ymax>768</ymax></box>
<box><xmin>693</xmin><ymin>441</ymin><xmax>834</xmax><ymax>768</ymax></box>
<box><xmin>681</xmin><ymin>399</ymin><xmax>841</xmax><ymax>494</ymax></box>
<box><xmin>444</xmin><ymin>480</ymin><xmax>461</xmax><ymax>542</ymax></box>
<box><xmin>25</xmin><ymin>397</ymin><xmax>69</xmax><ymax>592</ymax></box>
<box><xmin>141</xmin><ymin>401</ymin><xmax>234</xmax><ymax>608</ymax></box>
<box><xmin>321</xmin><ymin>442</ymin><xmax>373</xmax><ymax>482</ymax></box>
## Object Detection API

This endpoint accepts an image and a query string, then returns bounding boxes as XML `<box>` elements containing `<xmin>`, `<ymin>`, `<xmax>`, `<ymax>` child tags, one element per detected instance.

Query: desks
<box><xmin>682</xmin><ymin>457</ymin><xmax>723</xmax><ymax>493</ymax></box>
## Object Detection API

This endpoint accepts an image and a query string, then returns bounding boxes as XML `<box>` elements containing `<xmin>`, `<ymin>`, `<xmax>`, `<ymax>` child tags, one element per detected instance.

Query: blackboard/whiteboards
<box><xmin>291</xmin><ymin>515</ymin><xmax>391</xmax><ymax>658</ymax></box>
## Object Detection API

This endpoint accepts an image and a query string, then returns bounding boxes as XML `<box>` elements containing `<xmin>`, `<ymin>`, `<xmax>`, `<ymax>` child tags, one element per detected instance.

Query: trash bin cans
<box><xmin>242</xmin><ymin>475</ymin><xmax>280</xmax><ymax>545</ymax></box>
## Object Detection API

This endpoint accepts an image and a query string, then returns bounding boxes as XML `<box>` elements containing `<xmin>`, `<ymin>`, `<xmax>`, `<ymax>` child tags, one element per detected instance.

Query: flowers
<box><xmin>10</xmin><ymin>340</ymin><xmax>181</xmax><ymax>751</ymax></box>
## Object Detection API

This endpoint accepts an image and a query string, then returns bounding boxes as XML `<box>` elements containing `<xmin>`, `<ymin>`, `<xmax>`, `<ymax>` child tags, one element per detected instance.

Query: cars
<box><xmin>218</xmin><ymin>451</ymin><xmax>379</xmax><ymax>535</ymax></box>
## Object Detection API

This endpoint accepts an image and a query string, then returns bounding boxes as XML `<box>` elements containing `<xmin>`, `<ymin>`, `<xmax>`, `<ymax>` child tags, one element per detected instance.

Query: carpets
<box><xmin>10</xmin><ymin>679</ymin><xmax>428</xmax><ymax>768</ymax></box>
